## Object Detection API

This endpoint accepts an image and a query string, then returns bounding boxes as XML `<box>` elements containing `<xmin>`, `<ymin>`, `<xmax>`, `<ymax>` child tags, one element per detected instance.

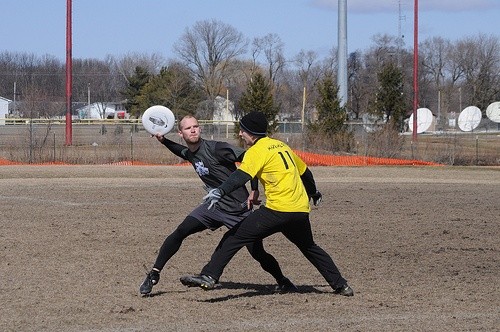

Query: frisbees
<box><xmin>140</xmin><ymin>104</ymin><xmax>177</xmax><ymax>137</ymax></box>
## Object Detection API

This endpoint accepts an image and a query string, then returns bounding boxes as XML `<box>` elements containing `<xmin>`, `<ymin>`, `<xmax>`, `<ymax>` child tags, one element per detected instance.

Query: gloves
<box><xmin>202</xmin><ymin>188</ymin><xmax>225</xmax><ymax>212</ymax></box>
<box><xmin>307</xmin><ymin>190</ymin><xmax>323</xmax><ymax>206</ymax></box>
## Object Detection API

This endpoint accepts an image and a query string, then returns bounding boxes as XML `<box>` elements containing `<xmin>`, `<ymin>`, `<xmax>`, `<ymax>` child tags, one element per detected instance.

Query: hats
<box><xmin>239</xmin><ymin>112</ymin><xmax>268</xmax><ymax>136</ymax></box>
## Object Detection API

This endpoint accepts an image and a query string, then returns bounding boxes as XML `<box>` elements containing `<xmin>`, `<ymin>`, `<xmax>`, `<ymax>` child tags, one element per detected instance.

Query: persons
<box><xmin>179</xmin><ymin>110</ymin><xmax>356</xmax><ymax>295</ymax></box>
<box><xmin>139</xmin><ymin>115</ymin><xmax>296</xmax><ymax>295</ymax></box>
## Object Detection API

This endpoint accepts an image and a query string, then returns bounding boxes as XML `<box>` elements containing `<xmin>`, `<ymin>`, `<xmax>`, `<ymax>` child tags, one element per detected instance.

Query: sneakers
<box><xmin>179</xmin><ymin>274</ymin><xmax>217</xmax><ymax>291</ymax></box>
<box><xmin>274</xmin><ymin>277</ymin><xmax>297</xmax><ymax>292</ymax></box>
<box><xmin>140</xmin><ymin>269</ymin><xmax>160</xmax><ymax>294</ymax></box>
<box><xmin>333</xmin><ymin>282</ymin><xmax>354</xmax><ymax>296</ymax></box>
